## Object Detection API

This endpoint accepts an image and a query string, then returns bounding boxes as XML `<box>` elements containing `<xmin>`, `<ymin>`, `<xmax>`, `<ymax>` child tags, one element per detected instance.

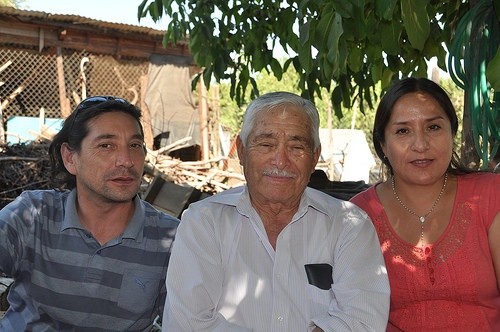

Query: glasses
<box><xmin>71</xmin><ymin>96</ymin><xmax>128</xmax><ymax>130</ymax></box>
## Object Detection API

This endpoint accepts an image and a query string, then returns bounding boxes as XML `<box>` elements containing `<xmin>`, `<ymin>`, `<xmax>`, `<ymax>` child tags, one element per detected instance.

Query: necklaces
<box><xmin>391</xmin><ymin>173</ymin><xmax>447</xmax><ymax>248</ymax></box>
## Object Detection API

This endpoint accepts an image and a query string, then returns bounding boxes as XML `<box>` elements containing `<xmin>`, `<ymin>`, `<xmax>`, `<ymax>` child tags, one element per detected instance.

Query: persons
<box><xmin>0</xmin><ymin>95</ymin><xmax>181</xmax><ymax>332</ymax></box>
<box><xmin>348</xmin><ymin>78</ymin><xmax>500</xmax><ymax>332</ymax></box>
<box><xmin>162</xmin><ymin>91</ymin><xmax>391</xmax><ymax>332</ymax></box>
